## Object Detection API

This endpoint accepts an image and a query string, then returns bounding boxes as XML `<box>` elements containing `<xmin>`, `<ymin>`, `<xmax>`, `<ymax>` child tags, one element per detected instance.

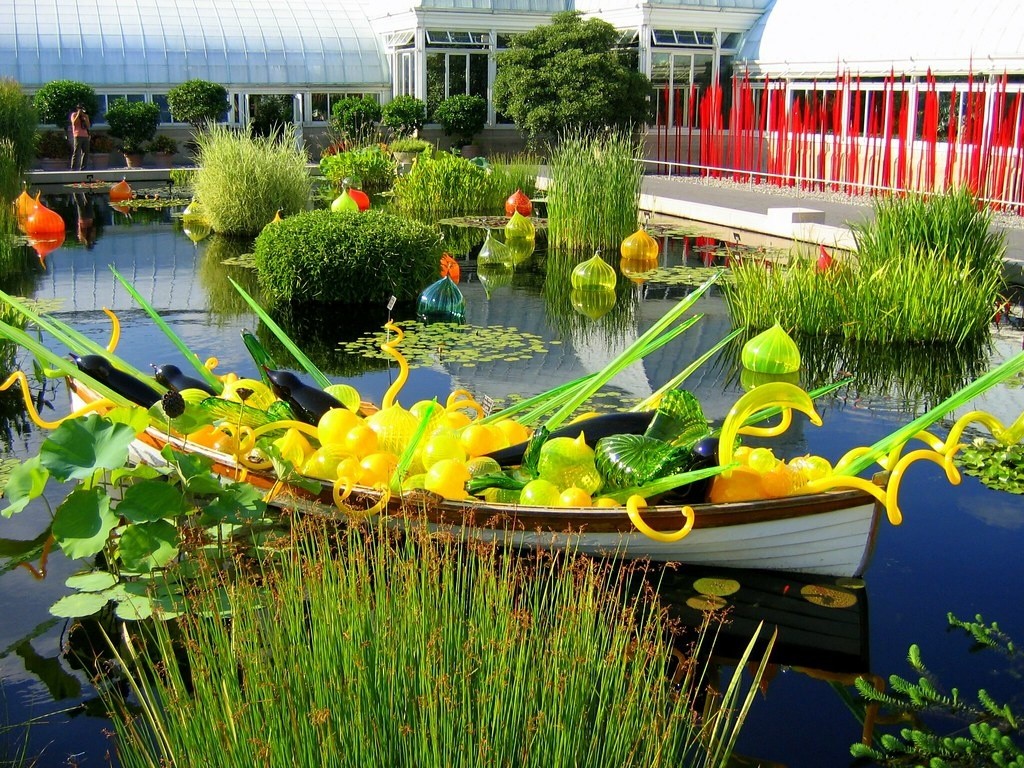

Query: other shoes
<box><xmin>80</xmin><ymin>168</ymin><xmax>87</xmax><ymax>171</ymax></box>
<box><xmin>71</xmin><ymin>168</ymin><xmax>75</xmax><ymax>170</ymax></box>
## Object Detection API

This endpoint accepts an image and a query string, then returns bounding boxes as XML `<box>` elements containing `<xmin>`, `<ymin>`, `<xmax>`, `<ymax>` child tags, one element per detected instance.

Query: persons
<box><xmin>73</xmin><ymin>192</ymin><xmax>98</xmax><ymax>250</ymax></box>
<box><xmin>69</xmin><ymin>102</ymin><xmax>90</xmax><ymax>171</ymax></box>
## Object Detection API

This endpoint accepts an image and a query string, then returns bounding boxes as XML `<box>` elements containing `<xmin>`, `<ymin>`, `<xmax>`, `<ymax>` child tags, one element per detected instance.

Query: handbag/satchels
<box><xmin>87</xmin><ymin>129</ymin><xmax>91</xmax><ymax>141</ymax></box>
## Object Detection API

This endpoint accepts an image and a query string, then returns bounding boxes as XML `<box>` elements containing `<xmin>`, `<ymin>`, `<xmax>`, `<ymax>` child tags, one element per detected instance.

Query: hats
<box><xmin>76</xmin><ymin>103</ymin><xmax>85</xmax><ymax>109</ymax></box>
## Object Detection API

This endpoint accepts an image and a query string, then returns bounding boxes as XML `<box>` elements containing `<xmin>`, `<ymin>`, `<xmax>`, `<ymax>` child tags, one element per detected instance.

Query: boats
<box><xmin>61</xmin><ymin>360</ymin><xmax>889</xmax><ymax>581</ymax></box>
<box><xmin>628</xmin><ymin>561</ymin><xmax>870</xmax><ymax>679</ymax></box>
